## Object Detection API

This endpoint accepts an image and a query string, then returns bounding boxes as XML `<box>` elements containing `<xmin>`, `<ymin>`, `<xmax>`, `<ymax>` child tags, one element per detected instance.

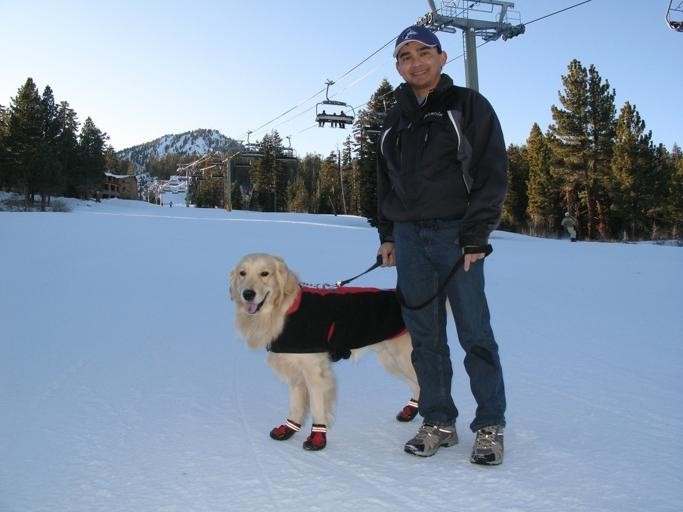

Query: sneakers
<box><xmin>471</xmin><ymin>426</ymin><xmax>504</xmax><ymax>465</ymax></box>
<box><xmin>405</xmin><ymin>423</ymin><xmax>459</xmax><ymax>458</ymax></box>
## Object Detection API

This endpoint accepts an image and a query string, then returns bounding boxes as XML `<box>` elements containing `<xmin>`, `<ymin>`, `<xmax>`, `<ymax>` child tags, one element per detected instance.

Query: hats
<box><xmin>393</xmin><ymin>25</ymin><xmax>441</xmax><ymax>57</ymax></box>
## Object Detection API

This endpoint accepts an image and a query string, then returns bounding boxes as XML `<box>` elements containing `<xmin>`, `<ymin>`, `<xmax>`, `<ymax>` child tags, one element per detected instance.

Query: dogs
<box><xmin>228</xmin><ymin>252</ymin><xmax>453</xmax><ymax>451</ymax></box>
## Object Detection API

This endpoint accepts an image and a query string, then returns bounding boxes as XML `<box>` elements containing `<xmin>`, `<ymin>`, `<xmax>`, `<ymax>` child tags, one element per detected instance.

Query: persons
<box><xmin>338</xmin><ymin>109</ymin><xmax>346</xmax><ymax>128</ymax></box>
<box><xmin>374</xmin><ymin>23</ymin><xmax>516</xmax><ymax>467</ymax></box>
<box><xmin>317</xmin><ymin>109</ymin><xmax>327</xmax><ymax>127</ymax></box>
<box><xmin>168</xmin><ymin>200</ymin><xmax>173</xmax><ymax>209</ymax></box>
<box><xmin>560</xmin><ymin>211</ymin><xmax>578</xmax><ymax>242</ymax></box>
<box><xmin>330</xmin><ymin>112</ymin><xmax>337</xmax><ymax>128</ymax></box>
